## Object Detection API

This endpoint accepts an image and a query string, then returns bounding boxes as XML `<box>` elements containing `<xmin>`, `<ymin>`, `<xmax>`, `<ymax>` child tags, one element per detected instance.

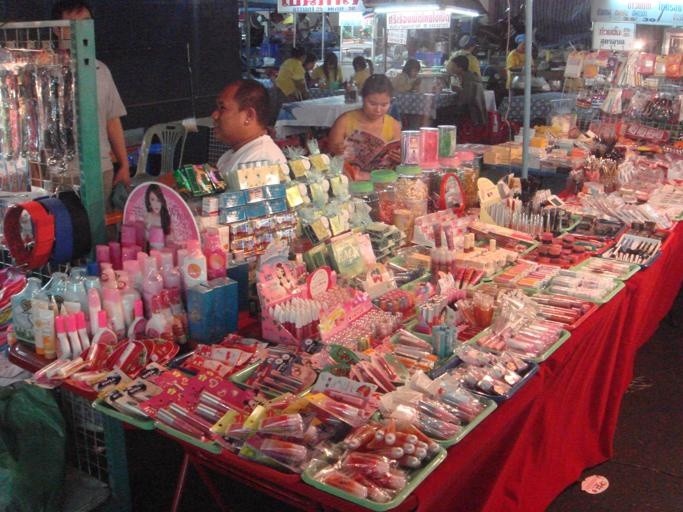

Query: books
<box><xmin>332</xmin><ymin>128</ymin><xmax>403</xmax><ymax>174</ymax></box>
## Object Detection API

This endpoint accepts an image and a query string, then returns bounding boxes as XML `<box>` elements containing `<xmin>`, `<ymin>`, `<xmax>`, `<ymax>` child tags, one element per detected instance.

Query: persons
<box><xmin>323</xmin><ymin>72</ymin><xmax>406</xmax><ymax>165</ymax></box>
<box><xmin>504</xmin><ymin>33</ymin><xmax>537</xmax><ymax>100</ymax></box>
<box><xmin>264</xmin><ymin>39</ymin><xmax>489</xmax><ymax>130</ymax></box>
<box><xmin>208</xmin><ymin>78</ymin><xmax>290</xmax><ymax>185</ymax></box>
<box><xmin>137</xmin><ymin>184</ymin><xmax>175</xmax><ymax>248</ymax></box>
<box><xmin>49</xmin><ymin>0</ymin><xmax>132</xmax><ymax>215</ymax></box>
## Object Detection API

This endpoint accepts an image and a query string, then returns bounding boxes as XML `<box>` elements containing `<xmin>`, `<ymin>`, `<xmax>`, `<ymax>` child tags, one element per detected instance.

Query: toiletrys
<box><xmin>10</xmin><ymin>236</ymin><xmax>208</xmax><ymax>363</ymax></box>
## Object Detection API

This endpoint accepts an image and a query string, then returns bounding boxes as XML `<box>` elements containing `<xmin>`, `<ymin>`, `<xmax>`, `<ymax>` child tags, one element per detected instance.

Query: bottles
<box><xmin>419</xmin><ymin>127</ymin><xmax>439</xmax><ymax>171</ymax></box>
<box><xmin>437</xmin><ymin>124</ymin><xmax>458</xmax><ymax>159</ymax></box>
<box><xmin>399</xmin><ymin>129</ymin><xmax>419</xmax><ymax>167</ymax></box>
<box><xmin>348</xmin><ymin>149</ymin><xmax>480</xmax><ymax>229</ymax></box>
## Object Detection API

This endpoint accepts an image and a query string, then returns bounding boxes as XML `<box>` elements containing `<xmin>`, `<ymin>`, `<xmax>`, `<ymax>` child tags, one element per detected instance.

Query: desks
<box><xmin>0</xmin><ymin>11</ymin><xmax>683</xmax><ymax>512</ymax></box>
